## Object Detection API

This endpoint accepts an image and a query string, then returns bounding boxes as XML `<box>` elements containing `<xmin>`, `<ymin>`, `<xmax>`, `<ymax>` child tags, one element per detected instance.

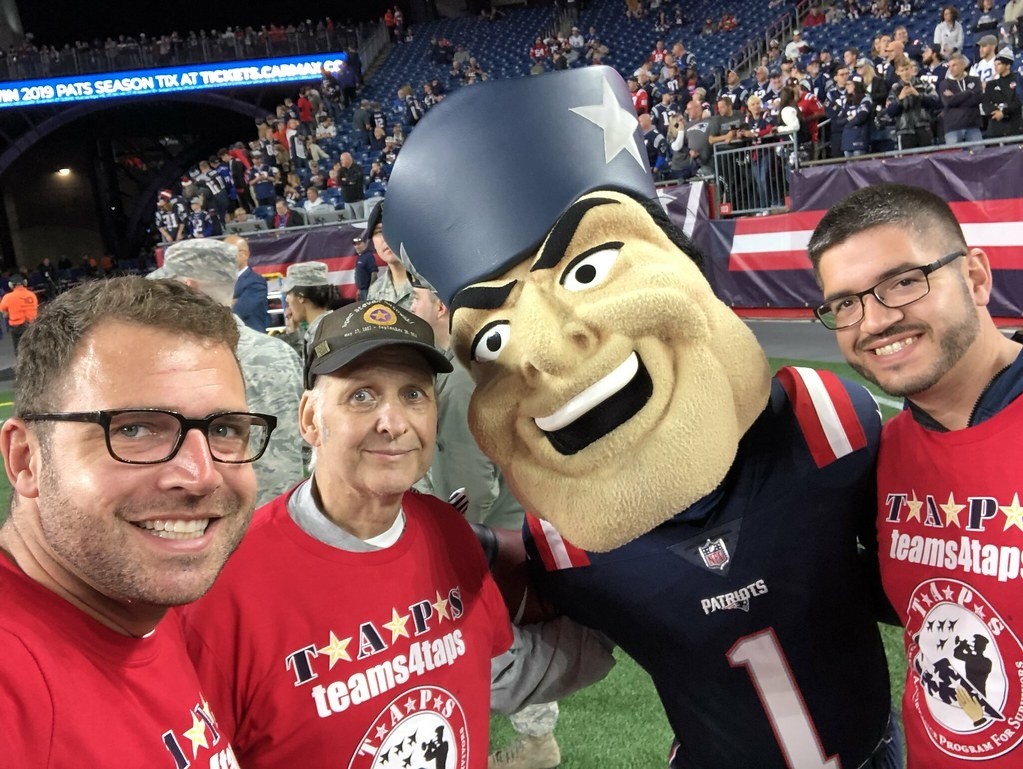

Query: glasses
<box><xmin>770</xmin><ymin>46</ymin><xmax>778</xmax><ymax>49</ymax></box>
<box><xmin>812</xmin><ymin>250</ymin><xmax>967</xmax><ymax>330</ymax></box>
<box><xmin>22</xmin><ymin>409</ymin><xmax>277</xmax><ymax>464</ymax></box>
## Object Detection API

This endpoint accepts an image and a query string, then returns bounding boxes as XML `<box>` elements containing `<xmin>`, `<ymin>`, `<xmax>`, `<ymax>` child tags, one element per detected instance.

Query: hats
<box><xmin>252</xmin><ymin>151</ymin><xmax>262</xmax><ymax>158</ymax></box>
<box><xmin>282</xmin><ymin>261</ymin><xmax>329</xmax><ymax>292</ymax></box>
<box><xmin>144</xmin><ymin>238</ymin><xmax>237</xmax><ymax>283</ymax></box>
<box><xmin>303</xmin><ymin>300</ymin><xmax>454</xmax><ymax>390</ymax></box>
<box><xmin>8</xmin><ymin>274</ymin><xmax>23</xmax><ymax>282</ymax></box>
<box><xmin>209</xmin><ymin>156</ymin><xmax>220</xmax><ymax>162</ymax></box>
<box><xmin>368</xmin><ymin>200</ymin><xmax>384</xmax><ymax>239</ymax></box>
<box><xmin>385</xmin><ymin>136</ymin><xmax>394</xmax><ymax>142</ymax></box>
<box><xmin>235</xmin><ymin>141</ymin><xmax>245</xmax><ymax>148</ymax></box>
<box><xmin>572</xmin><ymin>26</ymin><xmax>581</xmax><ymax>31</ymax></box>
<box><xmin>190</xmin><ymin>197</ymin><xmax>200</xmax><ymax>203</ymax></box>
<box><xmin>625</xmin><ymin>1</ymin><xmax>1019</xmax><ymax>107</ymax></box>
<box><xmin>406</xmin><ymin>269</ymin><xmax>427</xmax><ymax>288</ymax></box>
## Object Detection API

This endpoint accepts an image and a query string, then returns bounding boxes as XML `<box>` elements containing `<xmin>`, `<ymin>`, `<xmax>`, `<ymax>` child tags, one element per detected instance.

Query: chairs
<box><xmin>250</xmin><ymin>0</ymin><xmax>1023</xmax><ymax>228</ymax></box>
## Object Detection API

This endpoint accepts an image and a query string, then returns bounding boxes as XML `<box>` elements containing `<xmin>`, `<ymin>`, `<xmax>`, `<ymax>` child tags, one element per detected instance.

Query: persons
<box><xmin>381</xmin><ymin>65</ymin><xmax>1023</xmax><ymax>769</ymax></box>
<box><xmin>0</xmin><ymin>252</ymin><xmax>119</xmax><ymax>302</ymax></box>
<box><xmin>529</xmin><ymin>27</ymin><xmax>610</xmax><ymax>74</ymax></box>
<box><xmin>224</xmin><ymin>235</ymin><xmax>268</xmax><ymax>334</ymax></box>
<box><xmin>0</xmin><ymin>277</ymin><xmax>257</xmax><ymax>769</ymax></box>
<box><xmin>139</xmin><ymin>245</ymin><xmax>157</xmax><ymax>268</ymax></box>
<box><xmin>352</xmin><ymin>237</ymin><xmax>379</xmax><ymax>303</ymax></box>
<box><xmin>155</xmin><ymin>39</ymin><xmax>487</xmax><ymax>242</ymax></box>
<box><xmin>173</xmin><ymin>298</ymin><xmax>617</xmax><ymax>769</ymax></box>
<box><xmin>808</xmin><ymin>182</ymin><xmax>1022</xmax><ymax>769</ymax></box>
<box><xmin>0</xmin><ymin>16</ymin><xmax>385</xmax><ymax>81</ymax></box>
<box><xmin>625</xmin><ymin>0</ymin><xmax>1023</xmax><ymax>181</ymax></box>
<box><xmin>480</xmin><ymin>7</ymin><xmax>506</xmax><ymax>23</ymax></box>
<box><xmin>272</xmin><ymin>200</ymin><xmax>561</xmax><ymax>768</ymax></box>
<box><xmin>0</xmin><ymin>275</ymin><xmax>38</xmax><ymax>355</ymax></box>
<box><xmin>144</xmin><ymin>239</ymin><xmax>304</xmax><ymax>508</ymax></box>
<box><xmin>385</xmin><ymin>6</ymin><xmax>404</xmax><ymax>44</ymax></box>
